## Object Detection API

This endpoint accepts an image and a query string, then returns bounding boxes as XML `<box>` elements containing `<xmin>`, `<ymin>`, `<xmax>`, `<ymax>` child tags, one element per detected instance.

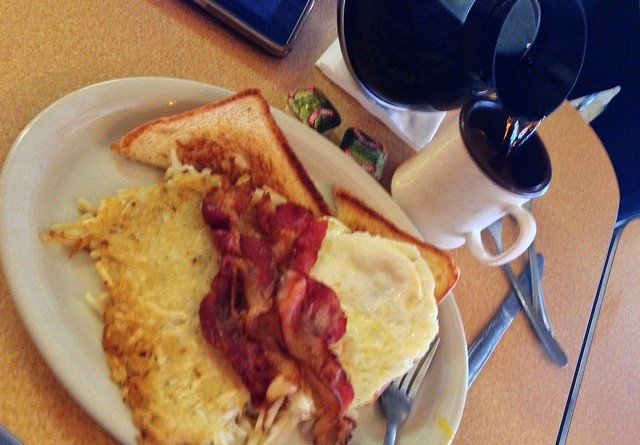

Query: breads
<box><xmin>334</xmin><ymin>185</ymin><xmax>460</xmax><ymax>303</ymax></box>
<box><xmin>110</xmin><ymin>87</ymin><xmax>330</xmax><ymax>216</ymax></box>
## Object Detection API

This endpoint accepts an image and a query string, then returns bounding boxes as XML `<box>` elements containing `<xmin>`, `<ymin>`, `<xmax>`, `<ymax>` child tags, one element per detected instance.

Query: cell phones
<box><xmin>187</xmin><ymin>0</ymin><xmax>317</xmax><ymax>57</ymax></box>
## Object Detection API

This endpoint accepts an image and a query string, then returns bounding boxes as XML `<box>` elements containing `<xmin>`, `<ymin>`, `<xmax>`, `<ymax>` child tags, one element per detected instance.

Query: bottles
<box><xmin>338</xmin><ymin>1</ymin><xmax>587</xmax><ymax>131</ymax></box>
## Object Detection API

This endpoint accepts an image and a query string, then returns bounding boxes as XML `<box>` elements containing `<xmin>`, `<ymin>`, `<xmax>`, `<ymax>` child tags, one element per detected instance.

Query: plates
<box><xmin>0</xmin><ymin>77</ymin><xmax>468</xmax><ymax>444</ymax></box>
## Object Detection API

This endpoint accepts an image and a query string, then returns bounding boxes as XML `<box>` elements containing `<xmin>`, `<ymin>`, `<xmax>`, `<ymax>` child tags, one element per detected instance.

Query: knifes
<box><xmin>468</xmin><ymin>252</ymin><xmax>545</xmax><ymax>393</ymax></box>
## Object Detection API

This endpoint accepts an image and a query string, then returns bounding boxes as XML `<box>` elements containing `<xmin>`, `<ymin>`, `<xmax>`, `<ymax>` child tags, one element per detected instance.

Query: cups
<box><xmin>390</xmin><ymin>100</ymin><xmax>551</xmax><ymax>268</ymax></box>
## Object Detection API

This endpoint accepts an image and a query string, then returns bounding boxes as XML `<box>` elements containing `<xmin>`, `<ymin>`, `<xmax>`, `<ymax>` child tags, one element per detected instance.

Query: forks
<box><xmin>378</xmin><ymin>335</ymin><xmax>441</xmax><ymax>444</ymax></box>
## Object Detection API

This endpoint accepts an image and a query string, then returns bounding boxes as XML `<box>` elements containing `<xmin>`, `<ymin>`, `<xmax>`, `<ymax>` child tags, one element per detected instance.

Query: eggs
<box><xmin>38</xmin><ymin>149</ymin><xmax>441</xmax><ymax>445</ymax></box>
